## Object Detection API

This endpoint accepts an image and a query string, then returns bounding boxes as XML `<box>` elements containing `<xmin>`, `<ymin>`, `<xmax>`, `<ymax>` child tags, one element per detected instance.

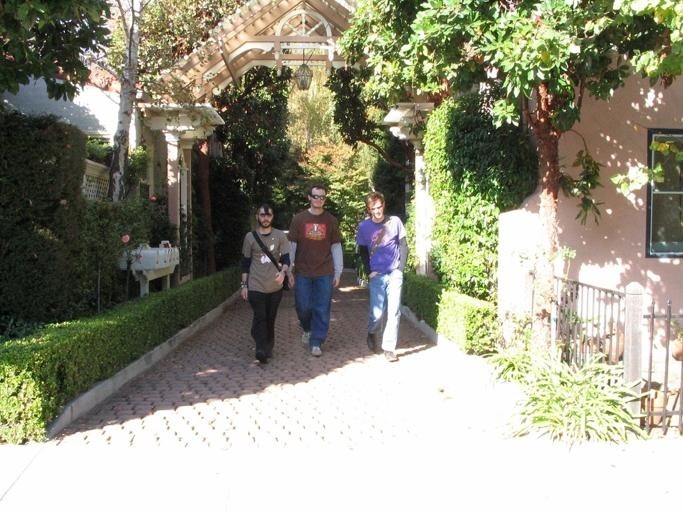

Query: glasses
<box><xmin>310</xmin><ymin>194</ymin><xmax>325</xmax><ymax>200</ymax></box>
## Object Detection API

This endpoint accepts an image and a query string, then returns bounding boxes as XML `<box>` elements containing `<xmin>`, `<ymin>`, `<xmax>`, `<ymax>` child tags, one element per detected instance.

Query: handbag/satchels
<box><xmin>280</xmin><ymin>269</ymin><xmax>289</xmax><ymax>290</ymax></box>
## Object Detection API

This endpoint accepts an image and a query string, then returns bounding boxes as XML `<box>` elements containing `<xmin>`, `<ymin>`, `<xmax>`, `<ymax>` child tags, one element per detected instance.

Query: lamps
<box><xmin>293</xmin><ymin>48</ymin><xmax>313</xmax><ymax>91</ymax></box>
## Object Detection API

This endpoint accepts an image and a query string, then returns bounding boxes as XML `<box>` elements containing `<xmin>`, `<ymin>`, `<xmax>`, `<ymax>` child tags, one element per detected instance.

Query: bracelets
<box><xmin>241</xmin><ymin>281</ymin><xmax>248</xmax><ymax>288</ymax></box>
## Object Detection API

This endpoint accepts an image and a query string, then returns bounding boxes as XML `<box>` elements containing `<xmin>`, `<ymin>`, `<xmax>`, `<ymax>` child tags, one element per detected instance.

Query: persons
<box><xmin>287</xmin><ymin>185</ymin><xmax>344</xmax><ymax>355</ymax></box>
<box><xmin>241</xmin><ymin>202</ymin><xmax>289</xmax><ymax>365</ymax></box>
<box><xmin>357</xmin><ymin>190</ymin><xmax>408</xmax><ymax>363</ymax></box>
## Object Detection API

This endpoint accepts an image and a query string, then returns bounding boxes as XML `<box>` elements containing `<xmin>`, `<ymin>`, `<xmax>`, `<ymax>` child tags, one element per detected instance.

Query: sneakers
<box><xmin>302</xmin><ymin>331</ymin><xmax>322</xmax><ymax>356</ymax></box>
<box><xmin>385</xmin><ymin>350</ymin><xmax>397</xmax><ymax>361</ymax></box>
<box><xmin>368</xmin><ymin>333</ymin><xmax>375</xmax><ymax>348</ymax></box>
<box><xmin>255</xmin><ymin>349</ymin><xmax>272</xmax><ymax>363</ymax></box>
